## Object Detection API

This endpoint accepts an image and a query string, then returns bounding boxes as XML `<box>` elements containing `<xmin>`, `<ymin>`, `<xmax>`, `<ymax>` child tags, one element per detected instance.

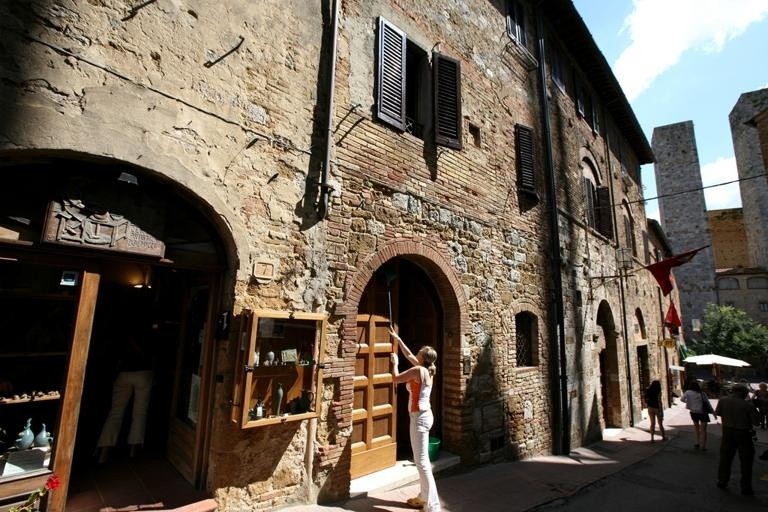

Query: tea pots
<box><xmin>298</xmin><ymin>389</ymin><xmax>314</xmax><ymax>411</ymax></box>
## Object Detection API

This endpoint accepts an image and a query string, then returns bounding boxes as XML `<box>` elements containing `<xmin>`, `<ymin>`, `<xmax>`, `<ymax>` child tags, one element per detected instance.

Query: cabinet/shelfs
<box><xmin>231</xmin><ymin>307</ymin><xmax>328</xmax><ymax>429</ymax></box>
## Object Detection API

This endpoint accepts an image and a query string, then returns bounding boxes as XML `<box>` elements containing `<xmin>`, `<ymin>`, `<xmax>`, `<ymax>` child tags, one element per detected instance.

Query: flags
<box><xmin>662</xmin><ymin>302</ymin><xmax>681</xmax><ymax>335</ymax></box>
<box><xmin>645</xmin><ymin>248</ymin><xmax>699</xmax><ymax>298</ymax></box>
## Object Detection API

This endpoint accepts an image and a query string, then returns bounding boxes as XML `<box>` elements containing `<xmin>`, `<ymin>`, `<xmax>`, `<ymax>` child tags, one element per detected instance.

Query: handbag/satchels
<box><xmin>702</xmin><ymin>399</ymin><xmax>713</xmax><ymax>414</ymax></box>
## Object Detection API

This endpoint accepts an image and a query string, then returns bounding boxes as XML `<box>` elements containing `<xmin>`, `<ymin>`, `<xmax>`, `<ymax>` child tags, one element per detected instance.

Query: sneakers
<box><xmin>408</xmin><ymin>494</ymin><xmax>426</xmax><ymax>508</ymax></box>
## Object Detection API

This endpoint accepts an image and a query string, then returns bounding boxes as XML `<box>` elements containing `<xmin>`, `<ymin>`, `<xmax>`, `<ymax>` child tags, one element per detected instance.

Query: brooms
<box><xmin>369</xmin><ymin>271</ymin><xmax>405</xmax><ymax>394</ymax></box>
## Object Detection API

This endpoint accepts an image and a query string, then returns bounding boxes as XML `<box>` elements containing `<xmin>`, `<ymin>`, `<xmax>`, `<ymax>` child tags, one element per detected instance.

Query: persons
<box><xmin>643</xmin><ymin>379</ymin><xmax>666</xmax><ymax>444</ymax></box>
<box><xmin>714</xmin><ymin>382</ymin><xmax>762</xmax><ymax>497</ymax></box>
<box><xmin>94</xmin><ymin>328</ymin><xmax>157</xmax><ymax>466</ymax></box>
<box><xmin>680</xmin><ymin>380</ymin><xmax>717</xmax><ymax>451</ymax></box>
<box><xmin>752</xmin><ymin>382</ymin><xmax>768</xmax><ymax>431</ymax></box>
<box><xmin>384</xmin><ymin>322</ymin><xmax>443</xmax><ymax>512</ymax></box>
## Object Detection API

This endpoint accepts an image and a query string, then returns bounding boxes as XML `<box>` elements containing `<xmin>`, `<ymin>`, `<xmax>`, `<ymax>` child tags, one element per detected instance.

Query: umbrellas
<box><xmin>682</xmin><ymin>352</ymin><xmax>754</xmax><ymax>377</ymax></box>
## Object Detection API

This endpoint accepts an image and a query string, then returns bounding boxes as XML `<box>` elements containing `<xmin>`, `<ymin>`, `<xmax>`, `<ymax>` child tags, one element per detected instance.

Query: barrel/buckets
<box><xmin>429</xmin><ymin>436</ymin><xmax>441</xmax><ymax>461</ymax></box>
